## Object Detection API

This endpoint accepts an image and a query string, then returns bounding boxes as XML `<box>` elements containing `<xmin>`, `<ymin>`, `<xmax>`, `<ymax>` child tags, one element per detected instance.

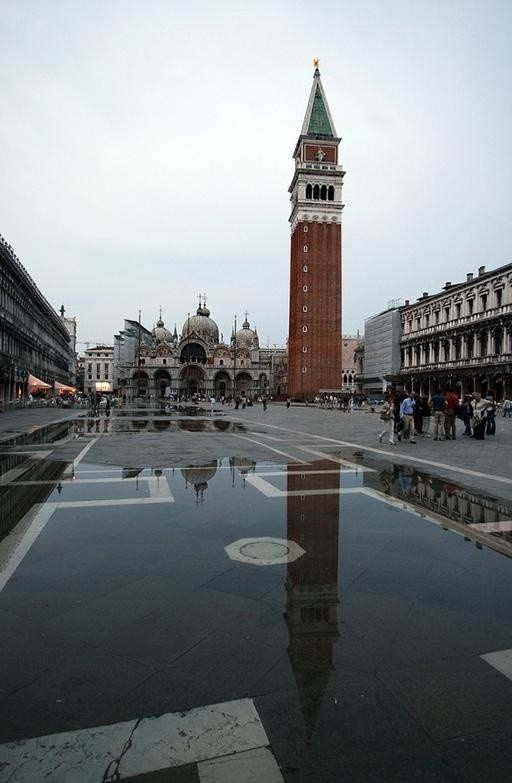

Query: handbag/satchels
<box><xmin>381</xmin><ymin>410</ymin><xmax>390</xmax><ymax>421</ymax></box>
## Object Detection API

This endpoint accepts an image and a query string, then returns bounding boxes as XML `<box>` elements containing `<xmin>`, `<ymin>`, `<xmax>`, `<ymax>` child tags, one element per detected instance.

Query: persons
<box><xmin>56</xmin><ymin>396</ymin><xmax>75</xmax><ymax>408</ymax></box>
<box><xmin>89</xmin><ymin>392</ymin><xmax>111</xmax><ymax>410</ymax></box>
<box><xmin>378</xmin><ymin>387</ymin><xmax>512</xmax><ymax>445</ymax></box>
<box><xmin>314</xmin><ymin>392</ymin><xmax>365</xmax><ymax>412</ymax></box>
<box><xmin>287</xmin><ymin>396</ymin><xmax>290</xmax><ymax>408</ymax></box>
<box><xmin>380</xmin><ymin>463</ymin><xmax>458</xmax><ymax>498</ymax></box>
<box><xmin>262</xmin><ymin>396</ymin><xmax>267</xmax><ymax>411</ymax></box>
<box><xmin>174</xmin><ymin>393</ymin><xmax>246</xmax><ymax>411</ymax></box>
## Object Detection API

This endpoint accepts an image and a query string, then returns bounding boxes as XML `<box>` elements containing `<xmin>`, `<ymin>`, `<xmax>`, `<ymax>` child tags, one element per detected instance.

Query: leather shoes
<box><xmin>378</xmin><ymin>431</ymin><xmax>496</xmax><ymax>446</ymax></box>
